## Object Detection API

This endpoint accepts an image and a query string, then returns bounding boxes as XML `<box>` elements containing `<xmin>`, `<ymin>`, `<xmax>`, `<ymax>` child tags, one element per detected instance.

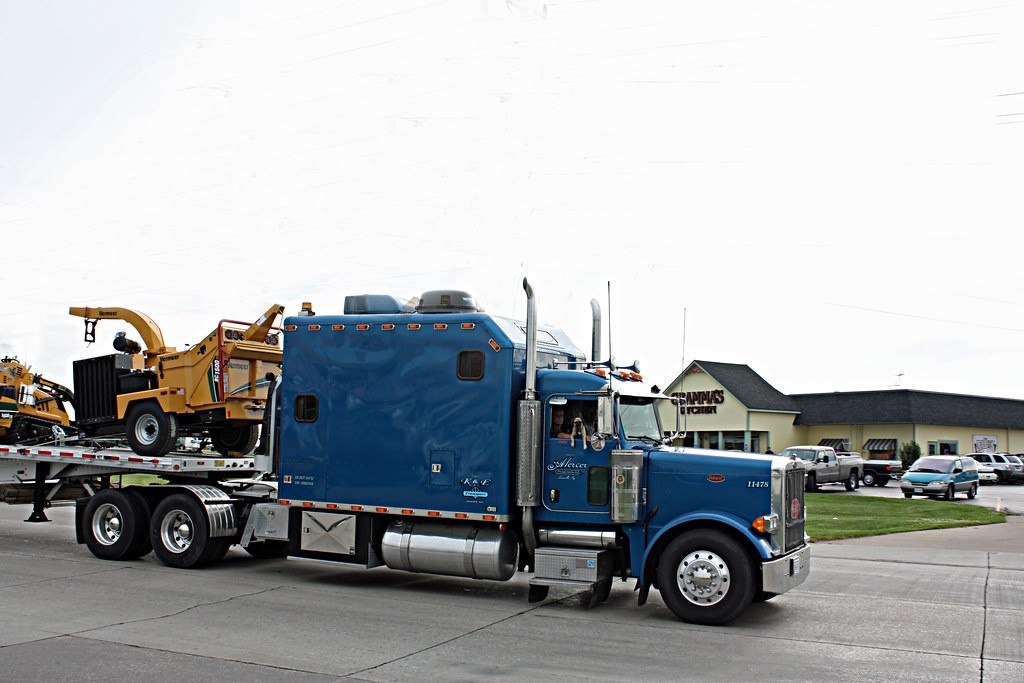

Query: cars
<box><xmin>966</xmin><ymin>452</ymin><xmax>1024</xmax><ymax>483</ymax></box>
<box><xmin>974</xmin><ymin>459</ymin><xmax>999</xmax><ymax>486</ymax></box>
<box><xmin>900</xmin><ymin>455</ymin><xmax>980</xmax><ymax>500</ymax></box>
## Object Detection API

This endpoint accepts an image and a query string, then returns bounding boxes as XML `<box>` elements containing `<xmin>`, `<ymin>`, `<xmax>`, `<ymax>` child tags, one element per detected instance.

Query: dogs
<box><xmin>570</xmin><ymin>416</ymin><xmax>588</xmax><ymax>450</ymax></box>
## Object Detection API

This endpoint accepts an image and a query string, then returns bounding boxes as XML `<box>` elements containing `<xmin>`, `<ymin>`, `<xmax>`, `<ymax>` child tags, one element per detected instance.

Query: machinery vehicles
<box><xmin>0</xmin><ymin>355</ymin><xmax>77</xmax><ymax>445</ymax></box>
<box><xmin>70</xmin><ymin>304</ymin><xmax>286</xmax><ymax>457</ymax></box>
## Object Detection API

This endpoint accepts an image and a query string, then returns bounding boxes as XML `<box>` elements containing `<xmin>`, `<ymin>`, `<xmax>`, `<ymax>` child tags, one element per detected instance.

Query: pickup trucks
<box><xmin>837</xmin><ymin>452</ymin><xmax>904</xmax><ymax>487</ymax></box>
<box><xmin>775</xmin><ymin>445</ymin><xmax>866</xmax><ymax>493</ymax></box>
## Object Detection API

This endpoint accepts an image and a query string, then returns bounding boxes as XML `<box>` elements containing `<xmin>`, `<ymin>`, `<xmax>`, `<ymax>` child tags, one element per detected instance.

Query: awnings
<box><xmin>862</xmin><ymin>440</ymin><xmax>895</xmax><ymax>450</ymax></box>
<box><xmin>818</xmin><ymin>439</ymin><xmax>844</xmax><ymax>449</ymax></box>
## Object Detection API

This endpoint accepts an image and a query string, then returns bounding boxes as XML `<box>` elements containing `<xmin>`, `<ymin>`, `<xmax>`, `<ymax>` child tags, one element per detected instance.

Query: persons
<box><xmin>766</xmin><ymin>447</ymin><xmax>774</xmax><ymax>454</ymax></box>
<box><xmin>551</xmin><ymin>409</ymin><xmax>590</xmax><ymax>439</ymax></box>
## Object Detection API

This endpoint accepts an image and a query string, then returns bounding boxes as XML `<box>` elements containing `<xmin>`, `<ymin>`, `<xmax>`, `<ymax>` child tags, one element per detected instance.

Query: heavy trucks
<box><xmin>0</xmin><ymin>278</ymin><xmax>817</xmax><ymax>625</ymax></box>
